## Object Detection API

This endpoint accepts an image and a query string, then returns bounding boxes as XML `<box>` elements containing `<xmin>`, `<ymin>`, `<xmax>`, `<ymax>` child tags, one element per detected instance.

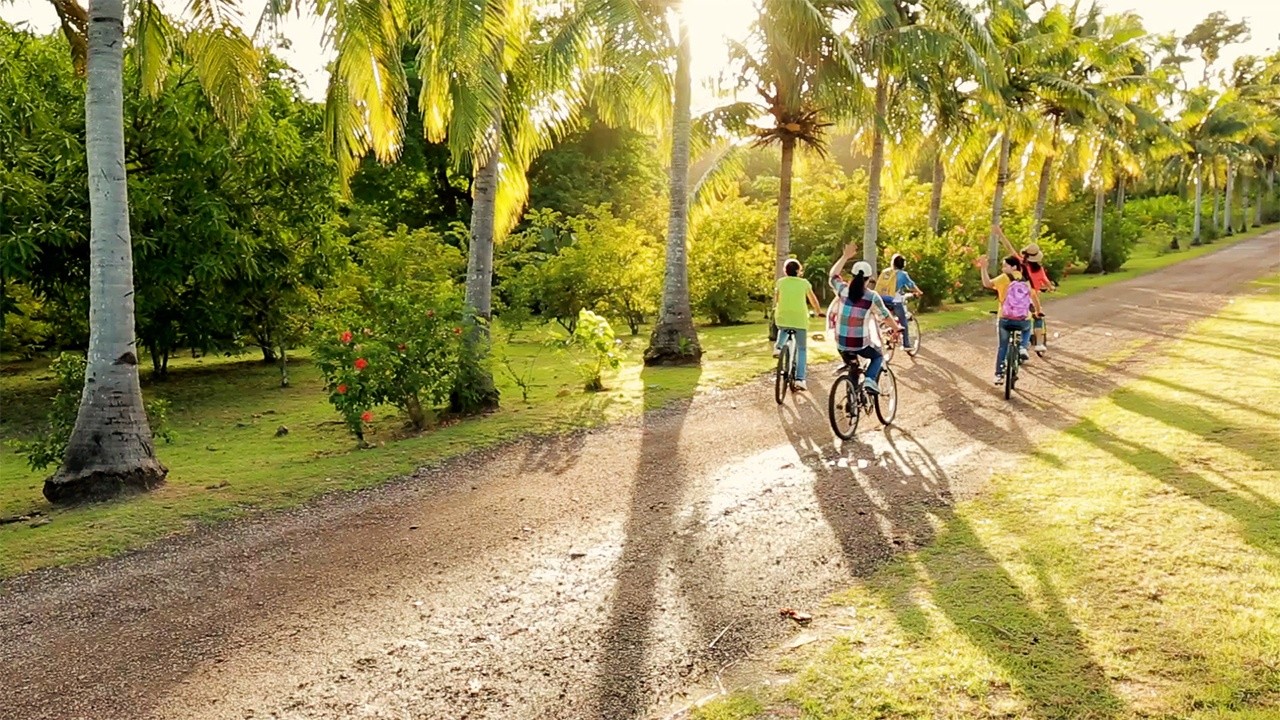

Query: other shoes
<box><xmin>863</xmin><ymin>380</ymin><xmax>881</xmax><ymax>393</ymax></box>
<box><xmin>995</xmin><ymin>374</ymin><xmax>1004</xmax><ymax>384</ymax></box>
<box><xmin>1019</xmin><ymin>349</ymin><xmax>1029</xmax><ymax>360</ymax></box>
<box><xmin>773</xmin><ymin>347</ymin><xmax>780</xmax><ymax>356</ymax></box>
<box><xmin>1034</xmin><ymin>345</ymin><xmax>1046</xmax><ymax>352</ymax></box>
<box><xmin>901</xmin><ymin>346</ymin><xmax>913</xmax><ymax>351</ymax></box>
<box><xmin>795</xmin><ymin>380</ymin><xmax>807</xmax><ymax>390</ymax></box>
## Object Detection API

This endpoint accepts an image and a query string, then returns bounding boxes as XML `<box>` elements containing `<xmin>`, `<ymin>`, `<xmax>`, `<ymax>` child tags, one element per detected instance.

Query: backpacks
<box><xmin>874</xmin><ymin>269</ymin><xmax>900</xmax><ymax>297</ymax></box>
<box><xmin>1001</xmin><ymin>274</ymin><xmax>1031</xmax><ymax>320</ymax></box>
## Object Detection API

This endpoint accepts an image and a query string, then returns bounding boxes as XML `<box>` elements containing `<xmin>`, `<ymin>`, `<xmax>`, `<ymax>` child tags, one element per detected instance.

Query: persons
<box><xmin>773</xmin><ymin>259</ymin><xmax>823</xmax><ymax>391</ymax></box>
<box><xmin>978</xmin><ymin>255</ymin><xmax>1045</xmax><ymax>385</ymax></box>
<box><xmin>881</xmin><ymin>254</ymin><xmax>923</xmax><ymax>351</ymax></box>
<box><xmin>826</xmin><ymin>240</ymin><xmax>904</xmax><ymax>419</ymax></box>
<box><xmin>998</xmin><ymin>229</ymin><xmax>1055</xmax><ymax>351</ymax></box>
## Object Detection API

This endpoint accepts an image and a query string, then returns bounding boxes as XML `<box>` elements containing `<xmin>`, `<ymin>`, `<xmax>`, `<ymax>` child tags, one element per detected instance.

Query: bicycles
<box><xmin>1028</xmin><ymin>285</ymin><xmax>1057</xmax><ymax>359</ymax></box>
<box><xmin>882</xmin><ymin>291</ymin><xmax>922</xmax><ymax>363</ymax></box>
<box><xmin>990</xmin><ymin>312</ymin><xmax>1042</xmax><ymax>402</ymax></box>
<box><xmin>773</xmin><ymin>309</ymin><xmax>827</xmax><ymax>405</ymax></box>
<box><xmin>828</xmin><ymin>322</ymin><xmax>906</xmax><ymax>441</ymax></box>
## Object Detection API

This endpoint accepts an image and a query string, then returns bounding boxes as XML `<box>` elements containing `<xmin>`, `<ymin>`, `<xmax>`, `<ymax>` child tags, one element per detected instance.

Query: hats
<box><xmin>852</xmin><ymin>262</ymin><xmax>872</xmax><ymax>277</ymax></box>
<box><xmin>1020</xmin><ymin>244</ymin><xmax>1043</xmax><ymax>262</ymax></box>
